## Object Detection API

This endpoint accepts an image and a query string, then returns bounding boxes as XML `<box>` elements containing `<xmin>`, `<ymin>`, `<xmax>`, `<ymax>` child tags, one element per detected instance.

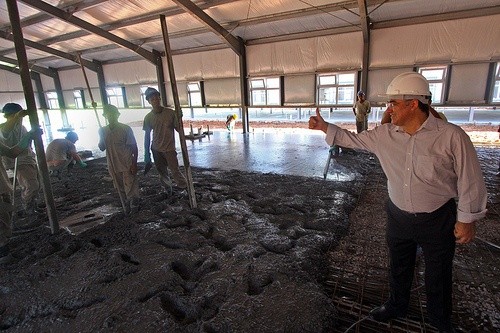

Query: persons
<box><xmin>142</xmin><ymin>87</ymin><xmax>189</xmax><ymax>194</ymax></box>
<box><xmin>0</xmin><ymin>103</ymin><xmax>44</xmax><ymax>231</ymax></box>
<box><xmin>307</xmin><ymin>71</ymin><xmax>488</xmax><ymax>333</ymax></box>
<box><xmin>46</xmin><ymin>132</ymin><xmax>87</xmax><ymax>175</ymax></box>
<box><xmin>98</xmin><ymin>104</ymin><xmax>141</xmax><ymax>215</ymax></box>
<box><xmin>225</xmin><ymin>113</ymin><xmax>238</xmax><ymax>131</ymax></box>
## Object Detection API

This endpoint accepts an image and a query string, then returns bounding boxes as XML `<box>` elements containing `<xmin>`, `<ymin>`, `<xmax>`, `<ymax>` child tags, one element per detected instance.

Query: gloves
<box><xmin>144</xmin><ymin>153</ymin><xmax>151</xmax><ymax>164</ymax></box>
<box><xmin>78</xmin><ymin>162</ymin><xmax>87</xmax><ymax>167</ymax></box>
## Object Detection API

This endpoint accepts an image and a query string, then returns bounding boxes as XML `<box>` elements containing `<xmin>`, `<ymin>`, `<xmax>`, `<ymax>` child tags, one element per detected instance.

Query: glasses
<box><xmin>386</xmin><ymin>99</ymin><xmax>410</xmax><ymax>108</ymax></box>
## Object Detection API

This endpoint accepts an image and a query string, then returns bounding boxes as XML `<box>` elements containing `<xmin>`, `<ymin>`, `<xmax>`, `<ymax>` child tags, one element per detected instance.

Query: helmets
<box><xmin>232</xmin><ymin>114</ymin><xmax>237</xmax><ymax>119</ymax></box>
<box><xmin>357</xmin><ymin>90</ymin><xmax>365</xmax><ymax>96</ymax></box>
<box><xmin>102</xmin><ymin>104</ymin><xmax>120</xmax><ymax>115</ymax></box>
<box><xmin>144</xmin><ymin>87</ymin><xmax>160</xmax><ymax>100</ymax></box>
<box><xmin>1</xmin><ymin>103</ymin><xmax>23</xmax><ymax>118</ymax></box>
<box><xmin>378</xmin><ymin>70</ymin><xmax>432</xmax><ymax>96</ymax></box>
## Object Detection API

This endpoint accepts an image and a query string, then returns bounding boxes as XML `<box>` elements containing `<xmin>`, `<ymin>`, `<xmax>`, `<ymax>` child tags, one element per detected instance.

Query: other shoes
<box><xmin>29</xmin><ymin>208</ymin><xmax>45</xmax><ymax>216</ymax></box>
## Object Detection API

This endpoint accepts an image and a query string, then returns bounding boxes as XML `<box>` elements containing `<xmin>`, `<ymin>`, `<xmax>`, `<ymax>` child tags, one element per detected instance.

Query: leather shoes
<box><xmin>431</xmin><ymin>318</ymin><xmax>467</xmax><ymax>333</ymax></box>
<box><xmin>369</xmin><ymin>301</ymin><xmax>409</xmax><ymax>318</ymax></box>
